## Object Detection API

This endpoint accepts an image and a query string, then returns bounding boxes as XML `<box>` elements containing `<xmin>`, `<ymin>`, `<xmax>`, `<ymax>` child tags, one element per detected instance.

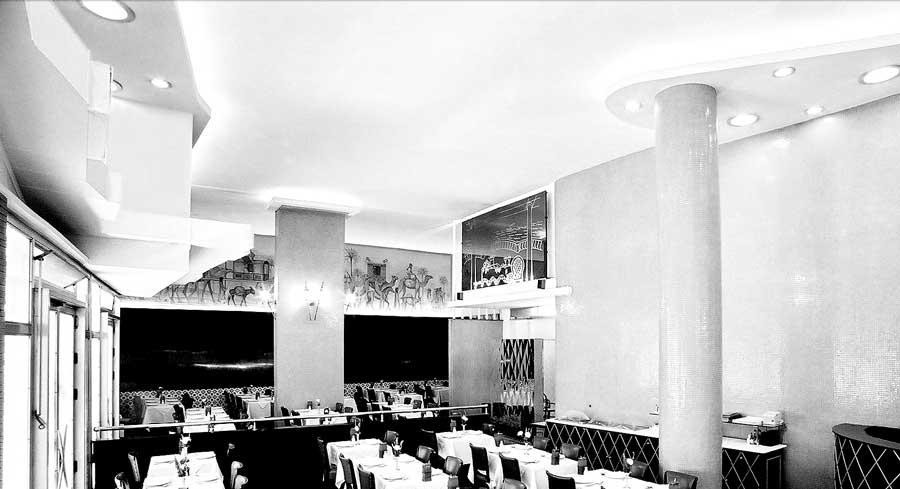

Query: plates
<box><xmin>147</xmin><ymin>450</ymin><xmax>221</xmax><ymax>487</ymax></box>
<box><xmin>330</xmin><ymin>438</ymin><xmax>380</xmax><ymax>467</ymax></box>
<box><xmin>242</xmin><ymin>390</ymin><xmax>425</xmax><ymax>419</ymax></box>
<box><xmin>147</xmin><ymin>396</ymin><xmax>229</xmax><ymax>430</ymax></box>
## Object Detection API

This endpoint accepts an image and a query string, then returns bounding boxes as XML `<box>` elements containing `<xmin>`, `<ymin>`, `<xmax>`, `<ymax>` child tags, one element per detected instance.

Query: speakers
<box><xmin>537</xmin><ymin>280</ymin><xmax>545</xmax><ymax>289</ymax></box>
<box><xmin>457</xmin><ymin>293</ymin><xmax>463</xmax><ymax>300</ymax></box>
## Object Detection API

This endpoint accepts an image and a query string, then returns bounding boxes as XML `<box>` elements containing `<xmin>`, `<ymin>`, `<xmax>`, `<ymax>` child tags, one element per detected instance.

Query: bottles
<box><xmin>669</xmin><ymin>477</ymin><xmax>680</xmax><ymax>489</ymax></box>
<box><xmin>551</xmin><ymin>445</ymin><xmax>561</xmax><ymax>466</ymax></box>
<box><xmin>421</xmin><ymin>464</ymin><xmax>431</xmax><ymax>481</ymax></box>
<box><xmin>447</xmin><ymin>476</ymin><xmax>459</xmax><ymax>488</ymax></box>
<box><xmin>449</xmin><ymin>420</ymin><xmax>457</xmax><ymax>431</ymax></box>
<box><xmin>378</xmin><ymin>440</ymin><xmax>389</xmax><ymax>458</ymax></box>
<box><xmin>496</xmin><ymin>432</ymin><xmax>505</xmax><ymax>447</ymax></box>
<box><xmin>577</xmin><ymin>455</ymin><xmax>588</xmax><ymax>475</ymax></box>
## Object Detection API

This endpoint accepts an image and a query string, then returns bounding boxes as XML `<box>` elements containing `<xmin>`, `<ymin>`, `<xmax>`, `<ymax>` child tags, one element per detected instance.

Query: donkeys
<box><xmin>228</xmin><ymin>287</ymin><xmax>255</xmax><ymax>307</ymax></box>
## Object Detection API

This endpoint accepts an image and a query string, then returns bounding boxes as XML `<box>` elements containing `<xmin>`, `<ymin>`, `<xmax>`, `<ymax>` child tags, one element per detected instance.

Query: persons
<box><xmin>406</xmin><ymin>263</ymin><xmax>416</xmax><ymax>279</ymax></box>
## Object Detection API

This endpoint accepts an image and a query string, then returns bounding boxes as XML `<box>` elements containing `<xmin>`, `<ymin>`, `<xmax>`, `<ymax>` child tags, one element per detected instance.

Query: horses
<box><xmin>366</xmin><ymin>286</ymin><xmax>395</xmax><ymax>307</ymax></box>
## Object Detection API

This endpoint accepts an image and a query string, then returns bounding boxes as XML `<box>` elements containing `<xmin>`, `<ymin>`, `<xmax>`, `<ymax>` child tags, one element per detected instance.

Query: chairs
<box><xmin>114</xmin><ymin>384</ymin><xmax>698</xmax><ymax>489</ymax></box>
